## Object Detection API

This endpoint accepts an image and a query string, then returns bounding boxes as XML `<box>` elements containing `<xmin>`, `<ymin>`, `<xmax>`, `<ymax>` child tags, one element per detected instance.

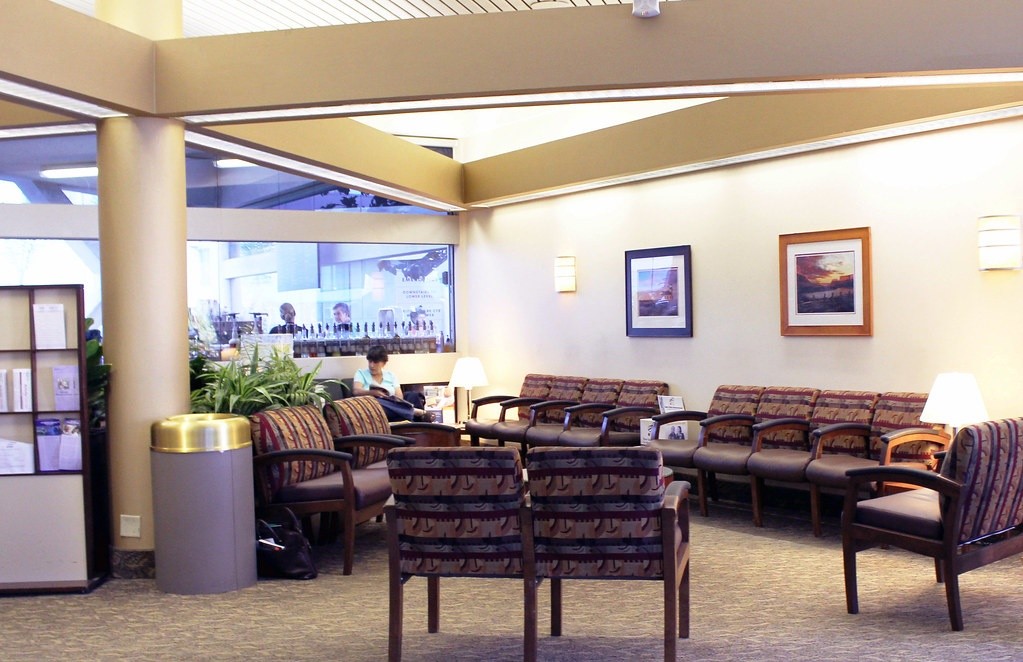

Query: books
<box><xmin>0</xmin><ymin>303</ymin><xmax>82</xmax><ymax>475</ymax></box>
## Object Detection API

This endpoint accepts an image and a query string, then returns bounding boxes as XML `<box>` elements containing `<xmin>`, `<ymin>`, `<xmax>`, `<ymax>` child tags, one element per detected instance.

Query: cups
<box><xmin>640</xmin><ymin>418</ymin><xmax>657</xmax><ymax>446</ymax></box>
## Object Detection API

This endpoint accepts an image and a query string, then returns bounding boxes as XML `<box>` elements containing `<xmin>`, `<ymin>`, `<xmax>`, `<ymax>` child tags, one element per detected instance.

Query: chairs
<box><xmin>840</xmin><ymin>417</ymin><xmax>1023</xmax><ymax>631</ymax></box>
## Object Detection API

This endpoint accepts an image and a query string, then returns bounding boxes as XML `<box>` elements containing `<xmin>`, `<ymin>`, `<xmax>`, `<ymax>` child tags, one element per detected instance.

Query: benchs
<box><xmin>248</xmin><ymin>373</ymin><xmax>950</xmax><ymax>662</ymax></box>
<box><xmin>311</xmin><ymin>378</ymin><xmax>355</xmax><ymax>403</ymax></box>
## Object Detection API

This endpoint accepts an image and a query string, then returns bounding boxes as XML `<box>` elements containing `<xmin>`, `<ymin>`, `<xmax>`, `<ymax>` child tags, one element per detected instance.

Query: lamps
<box><xmin>554</xmin><ymin>255</ymin><xmax>577</xmax><ymax>293</ymax></box>
<box><xmin>448</xmin><ymin>356</ymin><xmax>488</xmax><ymax>424</ymax></box>
<box><xmin>975</xmin><ymin>214</ymin><xmax>1023</xmax><ymax>270</ymax></box>
<box><xmin>919</xmin><ymin>372</ymin><xmax>990</xmax><ymax>437</ymax></box>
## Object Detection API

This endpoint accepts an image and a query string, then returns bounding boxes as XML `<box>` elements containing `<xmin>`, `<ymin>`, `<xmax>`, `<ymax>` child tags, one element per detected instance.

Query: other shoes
<box><xmin>418</xmin><ymin>410</ymin><xmax>436</xmax><ymax>421</ymax></box>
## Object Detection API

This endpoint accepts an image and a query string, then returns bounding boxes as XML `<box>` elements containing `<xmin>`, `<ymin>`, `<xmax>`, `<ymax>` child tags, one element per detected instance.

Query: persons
<box><xmin>333</xmin><ymin>302</ymin><xmax>356</xmax><ymax>332</ymax></box>
<box><xmin>668</xmin><ymin>425</ymin><xmax>684</xmax><ymax>440</ymax></box>
<box><xmin>269</xmin><ymin>303</ymin><xmax>308</xmax><ymax>337</ymax></box>
<box><xmin>379</xmin><ymin>310</ymin><xmax>400</xmax><ymax>344</ymax></box>
<box><xmin>352</xmin><ymin>346</ymin><xmax>436</xmax><ymax>423</ymax></box>
<box><xmin>406</xmin><ymin>310</ymin><xmax>433</xmax><ymax>335</ymax></box>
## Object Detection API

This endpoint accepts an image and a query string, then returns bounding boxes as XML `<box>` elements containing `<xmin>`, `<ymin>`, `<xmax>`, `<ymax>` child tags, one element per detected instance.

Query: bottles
<box><xmin>188</xmin><ymin>311</ymin><xmax>456</xmax><ymax>359</ymax></box>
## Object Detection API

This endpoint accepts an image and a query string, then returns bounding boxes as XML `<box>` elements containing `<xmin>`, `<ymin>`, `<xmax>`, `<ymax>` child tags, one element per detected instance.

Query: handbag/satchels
<box><xmin>257</xmin><ymin>507</ymin><xmax>317</xmax><ymax>581</ymax></box>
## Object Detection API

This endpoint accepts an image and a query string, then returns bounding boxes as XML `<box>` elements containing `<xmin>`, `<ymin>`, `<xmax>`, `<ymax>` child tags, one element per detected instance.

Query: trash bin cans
<box><xmin>149</xmin><ymin>413</ymin><xmax>258</xmax><ymax>595</ymax></box>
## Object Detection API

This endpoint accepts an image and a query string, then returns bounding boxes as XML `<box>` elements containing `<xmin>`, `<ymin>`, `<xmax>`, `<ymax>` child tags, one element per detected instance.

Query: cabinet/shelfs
<box><xmin>0</xmin><ymin>284</ymin><xmax>104</xmax><ymax>598</ymax></box>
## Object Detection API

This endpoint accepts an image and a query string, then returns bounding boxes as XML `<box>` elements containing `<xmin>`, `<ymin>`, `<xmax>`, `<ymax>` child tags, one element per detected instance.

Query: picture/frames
<box><xmin>778</xmin><ymin>227</ymin><xmax>873</xmax><ymax>337</ymax></box>
<box><xmin>625</xmin><ymin>245</ymin><xmax>693</xmax><ymax>338</ymax></box>
